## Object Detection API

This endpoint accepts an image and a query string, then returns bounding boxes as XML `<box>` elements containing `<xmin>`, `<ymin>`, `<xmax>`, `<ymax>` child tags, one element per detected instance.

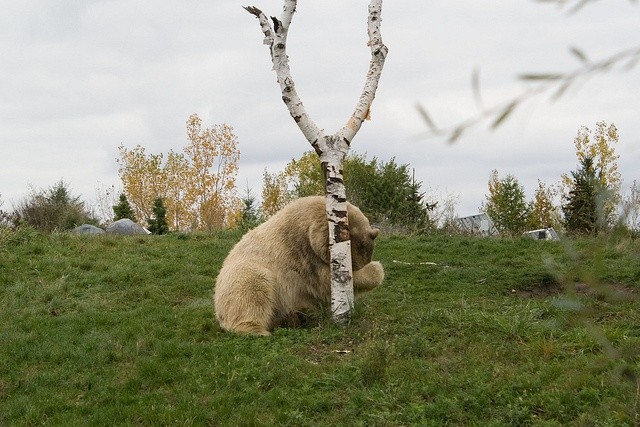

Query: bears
<box><xmin>212</xmin><ymin>195</ymin><xmax>385</xmax><ymax>339</ymax></box>
